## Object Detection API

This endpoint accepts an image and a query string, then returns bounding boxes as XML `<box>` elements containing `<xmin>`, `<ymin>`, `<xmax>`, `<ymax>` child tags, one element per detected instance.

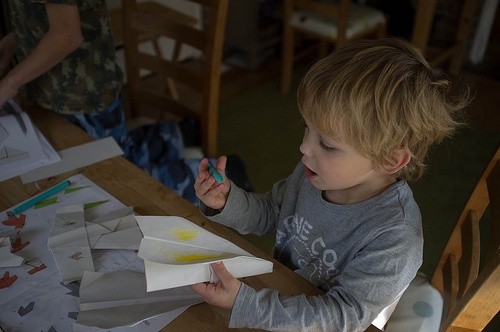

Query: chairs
<box><xmin>123</xmin><ymin>0</ymin><xmax>229</xmax><ymax>158</ymax></box>
<box><xmin>383</xmin><ymin>144</ymin><xmax>500</xmax><ymax>332</ymax></box>
<box><xmin>281</xmin><ymin>0</ymin><xmax>387</xmax><ymax>95</ymax></box>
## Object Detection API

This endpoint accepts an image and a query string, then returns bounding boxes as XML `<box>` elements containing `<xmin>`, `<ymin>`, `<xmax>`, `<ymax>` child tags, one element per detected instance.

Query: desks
<box><xmin>0</xmin><ymin>88</ymin><xmax>384</xmax><ymax>332</ymax></box>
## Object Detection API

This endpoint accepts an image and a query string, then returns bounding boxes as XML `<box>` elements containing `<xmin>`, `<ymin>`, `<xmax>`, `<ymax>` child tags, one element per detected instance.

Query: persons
<box><xmin>190</xmin><ymin>37</ymin><xmax>476</xmax><ymax>332</ymax></box>
<box><xmin>1</xmin><ymin>0</ymin><xmax>132</xmax><ymax>161</ymax></box>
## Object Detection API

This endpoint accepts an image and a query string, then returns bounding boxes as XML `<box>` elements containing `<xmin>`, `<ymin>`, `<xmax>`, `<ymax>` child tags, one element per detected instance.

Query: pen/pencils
<box><xmin>207</xmin><ymin>161</ymin><xmax>224</xmax><ymax>183</ymax></box>
<box><xmin>7</xmin><ymin>180</ymin><xmax>71</xmax><ymax>216</ymax></box>
